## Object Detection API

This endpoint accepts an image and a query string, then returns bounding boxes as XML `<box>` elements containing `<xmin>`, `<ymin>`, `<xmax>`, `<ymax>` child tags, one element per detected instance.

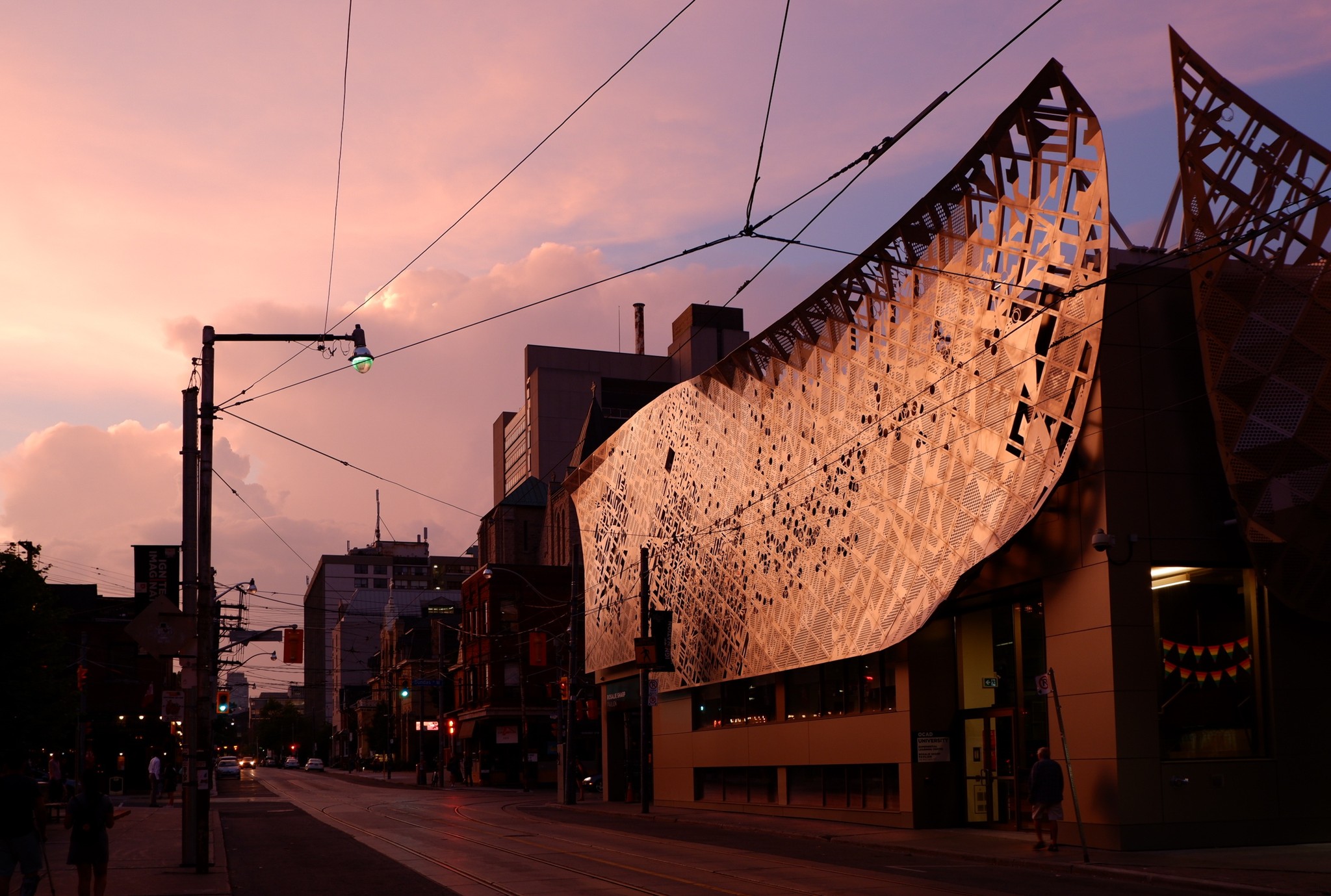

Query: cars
<box><xmin>258</xmin><ymin>757</ymin><xmax>276</xmax><ymax>766</ymax></box>
<box><xmin>215</xmin><ymin>756</ymin><xmax>241</xmax><ymax>780</ymax></box>
<box><xmin>304</xmin><ymin>758</ymin><xmax>324</xmax><ymax>772</ymax></box>
<box><xmin>583</xmin><ymin>772</ymin><xmax>605</xmax><ymax>792</ymax></box>
<box><xmin>239</xmin><ymin>757</ymin><xmax>255</xmax><ymax>769</ymax></box>
<box><xmin>285</xmin><ymin>756</ymin><xmax>301</xmax><ymax>769</ymax></box>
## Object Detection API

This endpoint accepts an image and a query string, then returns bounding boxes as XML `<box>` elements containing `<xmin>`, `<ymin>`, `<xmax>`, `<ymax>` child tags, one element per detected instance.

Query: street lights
<box><xmin>208</xmin><ymin>650</ymin><xmax>278</xmax><ymax>790</ymax></box>
<box><xmin>481</xmin><ymin>564</ymin><xmax>579</xmax><ymax>804</ymax></box>
<box><xmin>180</xmin><ymin>329</ymin><xmax>377</xmax><ymax>868</ymax></box>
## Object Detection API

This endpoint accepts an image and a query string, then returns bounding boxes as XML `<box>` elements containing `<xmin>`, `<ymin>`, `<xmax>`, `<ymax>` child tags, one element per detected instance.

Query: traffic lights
<box><xmin>401</xmin><ymin>680</ymin><xmax>408</xmax><ymax>697</ymax></box>
<box><xmin>291</xmin><ymin>746</ymin><xmax>295</xmax><ymax>750</ymax></box>
<box><xmin>82</xmin><ymin>720</ymin><xmax>94</xmax><ymax>741</ymax></box>
<box><xmin>77</xmin><ymin>665</ymin><xmax>89</xmax><ymax>692</ymax></box>
<box><xmin>215</xmin><ymin>691</ymin><xmax>229</xmax><ymax>714</ymax></box>
<box><xmin>447</xmin><ymin>719</ymin><xmax>455</xmax><ymax>736</ymax></box>
<box><xmin>559</xmin><ymin>676</ymin><xmax>570</xmax><ymax>700</ymax></box>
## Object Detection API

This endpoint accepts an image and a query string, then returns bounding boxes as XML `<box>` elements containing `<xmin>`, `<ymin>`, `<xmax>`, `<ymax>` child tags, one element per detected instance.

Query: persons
<box><xmin>0</xmin><ymin>744</ymin><xmax>49</xmax><ymax>896</ymax></box>
<box><xmin>148</xmin><ymin>756</ymin><xmax>176</xmax><ymax>807</ymax></box>
<box><xmin>448</xmin><ymin>752</ymin><xmax>460</xmax><ymax>787</ymax></box>
<box><xmin>462</xmin><ymin>751</ymin><xmax>473</xmax><ymax>786</ymax></box>
<box><xmin>63</xmin><ymin>769</ymin><xmax>114</xmax><ymax>896</ymax></box>
<box><xmin>48</xmin><ymin>752</ymin><xmax>62</xmax><ymax>803</ymax></box>
<box><xmin>1028</xmin><ymin>747</ymin><xmax>1064</xmax><ymax>851</ymax></box>
<box><xmin>575</xmin><ymin>756</ymin><xmax>584</xmax><ymax>801</ymax></box>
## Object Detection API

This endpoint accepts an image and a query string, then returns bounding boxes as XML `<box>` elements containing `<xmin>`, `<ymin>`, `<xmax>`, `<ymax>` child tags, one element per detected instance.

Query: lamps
<box><xmin>1152</xmin><ymin>573</ymin><xmax>1190</xmax><ymax>590</ymax></box>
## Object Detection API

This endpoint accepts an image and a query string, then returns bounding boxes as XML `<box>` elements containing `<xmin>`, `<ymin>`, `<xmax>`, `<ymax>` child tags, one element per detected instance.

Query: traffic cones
<box><xmin>626</xmin><ymin>783</ymin><xmax>636</xmax><ymax>803</ymax></box>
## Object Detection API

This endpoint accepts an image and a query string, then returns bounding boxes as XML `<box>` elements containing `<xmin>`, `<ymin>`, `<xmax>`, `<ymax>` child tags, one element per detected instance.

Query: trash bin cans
<box><xmin>415</xmin><ymin>764</ymin><xmax>426</xmax><ymax>785</ymax></box>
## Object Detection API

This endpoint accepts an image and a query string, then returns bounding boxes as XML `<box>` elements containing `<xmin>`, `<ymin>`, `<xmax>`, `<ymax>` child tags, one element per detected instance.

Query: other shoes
<box><xmin>149</xmin><ymin>805</ymin><xmax>161</xmax><ymax>808</ymax></box>
<box><xmin>577</xmin><ymin>798</ymin><xmax>584</xmax><ymax>801</ymax></box>
<box><xmin>451</xmin><ymin>785</ymin><xmax>456</xmax><ymax>787</ymax></box>
